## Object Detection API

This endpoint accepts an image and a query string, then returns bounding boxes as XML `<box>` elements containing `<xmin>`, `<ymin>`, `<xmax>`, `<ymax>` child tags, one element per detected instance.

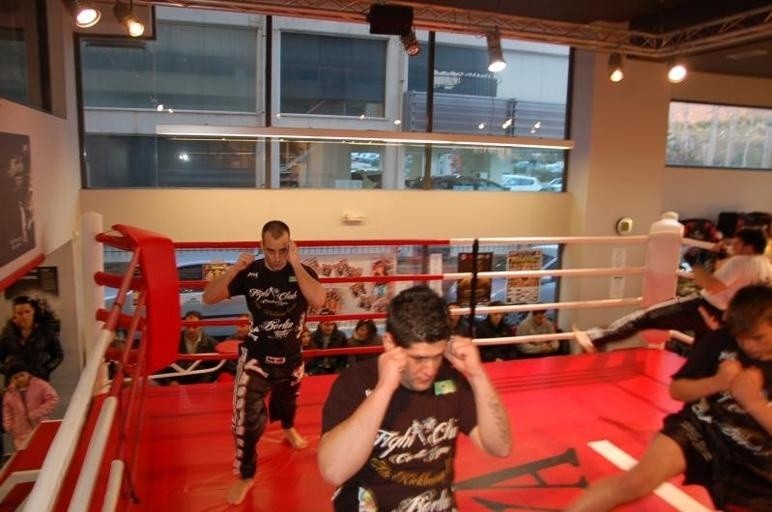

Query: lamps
<box><xmin>399</xmin><ymin>24</ymin><xmax>689</xmax><ymax>86</ymax></box>
<box><xmin>60</xmin><ymin>0</ymin><xmax>145</xmax><ymax>39</ymax></box>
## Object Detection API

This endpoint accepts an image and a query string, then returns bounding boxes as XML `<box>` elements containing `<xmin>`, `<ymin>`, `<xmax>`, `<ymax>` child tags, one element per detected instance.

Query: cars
<box><xmin>105</xmin><ymin>260</ymin><xmax>252</xmax><ymax>343</ymax></box>
<box><xmin>443</xmin><ymin>246</ymin><xmax>560</xmax><ymax>332</ymax></box>
<box><xmin>351</xmin><ymin>152</ymin><xmax>566</xmax><ymax>191</ymax></box>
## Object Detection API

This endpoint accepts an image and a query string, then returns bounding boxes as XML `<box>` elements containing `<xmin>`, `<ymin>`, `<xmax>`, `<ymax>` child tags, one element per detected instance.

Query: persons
<box><xmin>0</xmin><ymin>295</ymin><xmax>64</xmax><ymax>387</ymax></box>
<box><xmin>150</xmin><ymin>310</ymin><xmax>219</xmax><ymax>386</ymax></box>
<box><xmin>226</xmin><ymin>313</ymin><xmax>250</xmax><ymax>341</ymax></box>
<box><xmin>571</xmin><ymin>229</ymin><xmax>772</xmax><ymax>354</ymax></box>
<box><xmin>316</xmin><ymin>283</ymin><xmax>512</xmax><ymax>512</ymax></box>
<box><xmin>565</xmin><ymin>283</ymin><xmax>772</xmax><ymax>512</ymax></box>
<box><xmin>202</xmin><ymin>220</ymin><xmax>328</xmax><ymax>505</ymax></box>
<box><xmin>1</xmin><ymin>361</ymin><xmax>61</xmax><ymax>451</ymax></box>
<box><xmin>299</xmin><ymin>302</ymin><xmax>561</xmax><ymax>375</ymax></box>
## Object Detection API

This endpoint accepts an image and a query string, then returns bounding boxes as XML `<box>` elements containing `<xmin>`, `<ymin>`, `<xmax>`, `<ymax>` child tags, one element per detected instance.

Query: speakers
<box><xmin>368</xmin><ymin>4</ymin><xmax>413</xmax><ymax>35</ymax></box>
